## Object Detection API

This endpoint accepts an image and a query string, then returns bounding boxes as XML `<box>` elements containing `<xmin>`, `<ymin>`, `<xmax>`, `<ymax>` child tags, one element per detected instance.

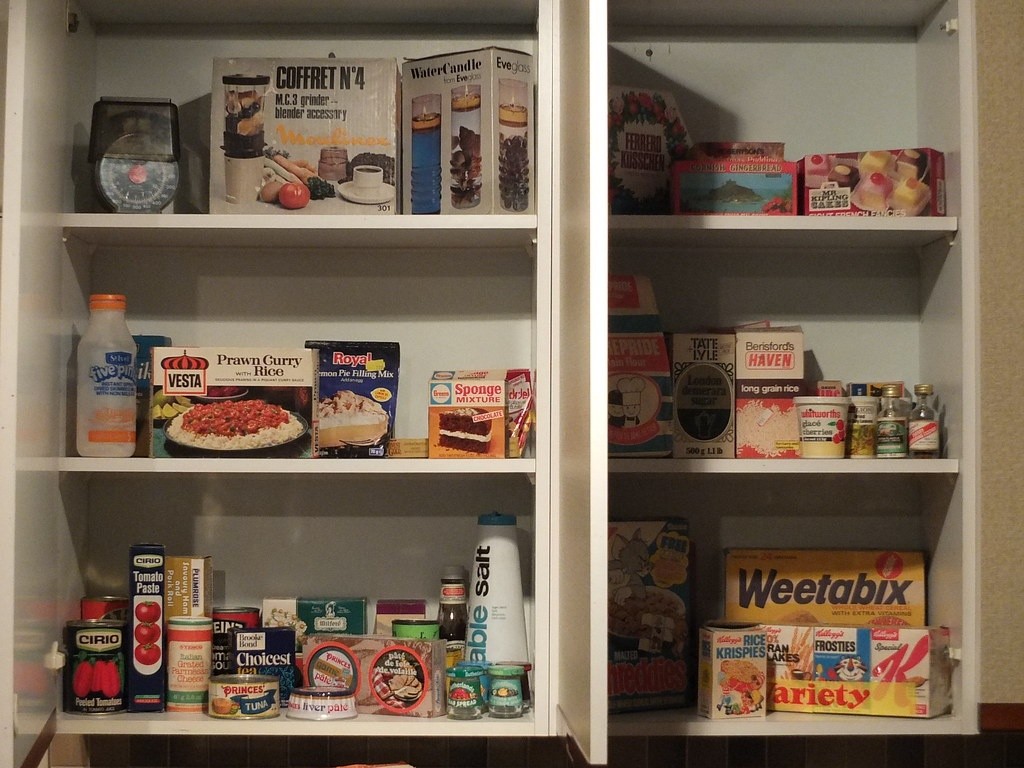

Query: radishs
<box><xmin>264</xmin><ymin>158</ymin><xmax>296</xmax><ymax>185</ymax></box>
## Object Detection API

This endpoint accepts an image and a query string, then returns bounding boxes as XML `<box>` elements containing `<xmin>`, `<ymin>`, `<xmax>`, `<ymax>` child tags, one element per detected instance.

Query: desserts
<box><xmin>437</xmin><ymin>408</ymin><xmax>493</xmax><ymax>453</ymax></box>
<box><xmin>804</xmin><ymin>149</ymin><xmax>932</xmax><ymax>216</ymax></box>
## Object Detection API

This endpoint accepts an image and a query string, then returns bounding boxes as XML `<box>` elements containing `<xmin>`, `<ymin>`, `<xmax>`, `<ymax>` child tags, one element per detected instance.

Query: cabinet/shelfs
<box><xmin>560</xmin><ymin>0</ymin><xmax>979</xmax><ymax>736</ymax></box>
<box><xmin>0</xmin><ymin>0</ymin><xmax>549</xmax><ymax>768</ymax></box>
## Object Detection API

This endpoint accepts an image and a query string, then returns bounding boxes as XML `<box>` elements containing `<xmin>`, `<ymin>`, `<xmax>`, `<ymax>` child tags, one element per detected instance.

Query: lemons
<box><xmin>152</xmin><ymin>388</ymin><xmax>194</xmax><ymax>418</ymax></box>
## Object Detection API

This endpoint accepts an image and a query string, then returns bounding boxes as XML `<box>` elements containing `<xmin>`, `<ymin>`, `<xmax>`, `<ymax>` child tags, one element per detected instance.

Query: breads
<box><xmin>212</xmin><ymin>697</ymin><xmax>231</xmax><ymax>714</ymax></box>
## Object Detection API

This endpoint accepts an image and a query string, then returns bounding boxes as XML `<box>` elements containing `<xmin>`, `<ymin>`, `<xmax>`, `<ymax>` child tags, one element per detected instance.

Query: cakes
<box><xmin>317</xmin><ymin>390</ymin><xmax>388</xmax><ymax>446</ymax></box>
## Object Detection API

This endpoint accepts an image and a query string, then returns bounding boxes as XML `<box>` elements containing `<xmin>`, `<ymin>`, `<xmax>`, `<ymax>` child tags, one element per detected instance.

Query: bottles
<box><xmin>909</xmin><ymin>384</ymin><xmax>940</xmax><ymax>458</ymax></box>
<box><xmin>75</xmin><ymin>294</ymin><xmax>137</xmax><ymax>458</ymax></box>
<box><xmin>875</xmin><ymin>385</ymin><xmax>908</xmax><ymax>458</ymax></box>
<box><xmin>437</xmin><ymin>566</ymin><xmax>469</xmax><ymax>671</ymax></box>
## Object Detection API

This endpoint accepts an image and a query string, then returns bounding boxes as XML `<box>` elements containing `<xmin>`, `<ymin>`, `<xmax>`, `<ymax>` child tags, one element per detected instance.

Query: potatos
<box><xmin>261</xmin><ymin>181</ymin><xmax>284</xmax><ymax>202</ymax></box>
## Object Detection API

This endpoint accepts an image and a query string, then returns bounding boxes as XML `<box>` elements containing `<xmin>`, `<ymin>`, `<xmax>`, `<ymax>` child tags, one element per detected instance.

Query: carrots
<box><xmin>273</xmin><ymin>154</ymin><xmax>317</xmax><ymax>186</ymax></box>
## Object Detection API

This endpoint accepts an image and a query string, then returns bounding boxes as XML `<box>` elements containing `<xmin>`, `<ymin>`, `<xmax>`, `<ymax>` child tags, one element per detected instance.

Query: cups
<box><xmin>353</xmin><ymin>165</ymin><xmax>383</xmax><ymax>197</ymax></box>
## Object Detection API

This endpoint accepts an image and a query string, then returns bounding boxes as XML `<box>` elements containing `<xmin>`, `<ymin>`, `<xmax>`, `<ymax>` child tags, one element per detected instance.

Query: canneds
<box><xmin>445</xmin><ymin>660</ymin><xmax>532</xmax><ymax>721</ymax></box>
<box><xmin>65</xmin><ymin>597</ymin><xmax>279</xmax><ymax>720</ymax></box>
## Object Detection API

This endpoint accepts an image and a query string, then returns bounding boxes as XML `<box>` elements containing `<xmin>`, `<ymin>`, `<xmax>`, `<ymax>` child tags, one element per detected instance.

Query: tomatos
<box><xmin>135</xmin><ymin>622</ymin><xmax>161</xmax><ymax>644</ymax></box>
<box><xmin>278</xmin><ymin>182</ymin><xmax>310</xmax><ymax>209</ymax></box>
<box><xmin>135</xmin><ymin>601</ymin><xmax>161</xmax><ymax>623</ymax></box>
<box><xmin>135</xmin><ymin>644</ymin><xmax>161</xmax><ymax>665</ymax></box>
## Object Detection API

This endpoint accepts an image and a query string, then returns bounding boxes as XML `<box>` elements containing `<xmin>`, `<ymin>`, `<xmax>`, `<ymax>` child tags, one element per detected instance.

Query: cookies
<box><xmin>389</xmin><ymin>674</ymin><xmax>423</xmax><ymax>699</ymax></box>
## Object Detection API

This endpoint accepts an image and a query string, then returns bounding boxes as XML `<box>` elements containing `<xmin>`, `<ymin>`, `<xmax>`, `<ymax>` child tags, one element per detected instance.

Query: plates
<box><xmin>162</xmin><ymin>408</ymin><xmax>308</xmax><ymax>452</ymax></box>
<box><xmin>196</xmin><ymin>387</ymin><xmax>249</xmax><ymax>403</ymax></box>
<box><xmin>338</xmin><ymin>181</ymin><xmax>395</xmax><ymax>204</ymax></box>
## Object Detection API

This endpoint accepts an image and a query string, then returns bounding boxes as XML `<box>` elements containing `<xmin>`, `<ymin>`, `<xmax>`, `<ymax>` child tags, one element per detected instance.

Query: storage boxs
<box><xmin>149</xmin><ymin>346</ymin><xmax>313</xmax><ymax>458</ymax></box>
<box><xmin>209</xmin><ymin>57</ymin><xmax>397</xmax><ymax>215</ymax></box>
<box><xmin>388</xmin><ymin>438</ymin><xmax>428</xmax><ymax>459</ymax></box>
<box><xmin>816</xmin><ymin>380</ymin><xmax>842</xmax><ymax>396</ymax></box>
<box><xmin>735</xmin><ymin>398</ymin><xmax>802</xmax><ymax>459</ymax></box>
<box><xmin>760</xmin><ymin>626</ymin><xmax>953</xmax><ymax>719</ymax></box>
<box><xmin>400</xmin><ymin>46</ymin><xmax>534</xmax><ymax>215</ymax></box>
<box><xmin>698</xmin><ymin>619</ymin><xmax>767</xmax><ymax>719</ymax></box>
<box><xmin>429</xmin><ymin>369</ymin><xmax>536</xmax><ymax>459</ymax></box>
<box><xmin>608</xmin><ymin>272</ymin><xmax>673</xmax><ymax>456</ymax></box>
<box><xmin>673</xmin><ymin>159</ymin><xmax>799</xmax><ymax>216</ymax></box>
<box><xmin>690</xmin><ymin>142</ymin><xmax>785</xmax><ymax>163</ymax></box>
<box><xmin>670</xmin><ymin>327</ymin><xmax>735</xmax><ymax>459</ymax></box>
<box><xmin>302</xmin><ymin>632</ymin><xmax>448</xmax><ymax>718</ymax></box>
<box><xmin>849</xmin><ymin>381</ymin><xmax>904</xmax><ymax>414</ymax></box>
<box><xmin>735</xmin><ymin>325</ymin><xmax>805</xmax><ymax>398</ymax></box>
<box><xmin>164</xmin><ymin>555</ymin><xmax>213</xmax><ymax>637</ymax></box>
<box><xmin>608</xmin><ymin>517</ymin><xmax>696</xmax><ymax>715</ymax></box>
<box><xmin>263</xmin><ymin>598</ymin><xmax>367</xmax><ymax>654</ymax></box>
<box><xmin>725</xmin><ymin>547</ymin><xmax>926</xmax><ymax>629</ymax></box>
<box><xmin>798</xmin><ymin>147</ymin><xmax>946</xmax><ymax>215</ymax></box>
